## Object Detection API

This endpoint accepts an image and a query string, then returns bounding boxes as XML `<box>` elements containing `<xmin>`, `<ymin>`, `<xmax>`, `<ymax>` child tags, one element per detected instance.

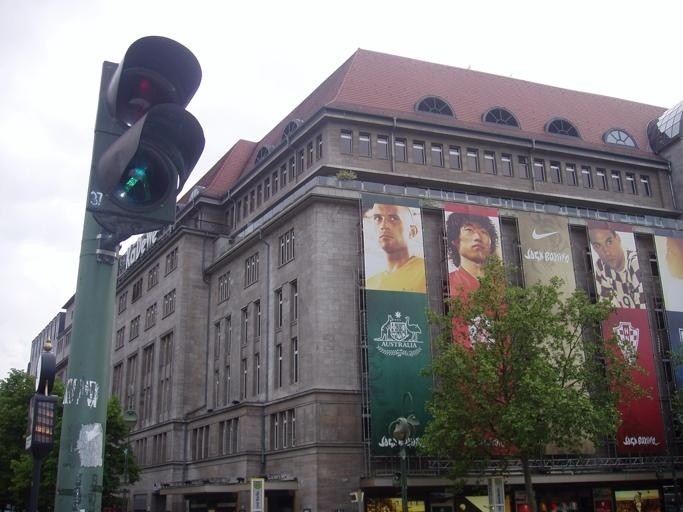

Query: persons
<box><xmin>444</xmin><ymin>212</ymin><xmax>508</xmax><ymax>298</ymax></box>
<box><xmin>588</xmin><ymin>227</ymin><xmax>647</xmax><ymax>311</ymax></box>
<box><xmin>364</xmin><ymin>202</ymin><xmax>426</xmax><ymax>293</ymax></box>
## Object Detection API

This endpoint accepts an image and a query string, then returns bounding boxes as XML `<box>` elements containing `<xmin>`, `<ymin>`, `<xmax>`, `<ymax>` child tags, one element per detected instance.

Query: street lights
<box><xmin>388</xmin><ymin>391</ymin><xmax>420</xmax><ymax>512</ymax></box>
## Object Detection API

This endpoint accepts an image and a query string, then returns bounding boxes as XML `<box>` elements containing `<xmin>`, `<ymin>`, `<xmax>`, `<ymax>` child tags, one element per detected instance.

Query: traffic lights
<box><xmin>98</xmin><ymin>30</ymin><xmax>206</xmax><ymax>217</ymax></box>
